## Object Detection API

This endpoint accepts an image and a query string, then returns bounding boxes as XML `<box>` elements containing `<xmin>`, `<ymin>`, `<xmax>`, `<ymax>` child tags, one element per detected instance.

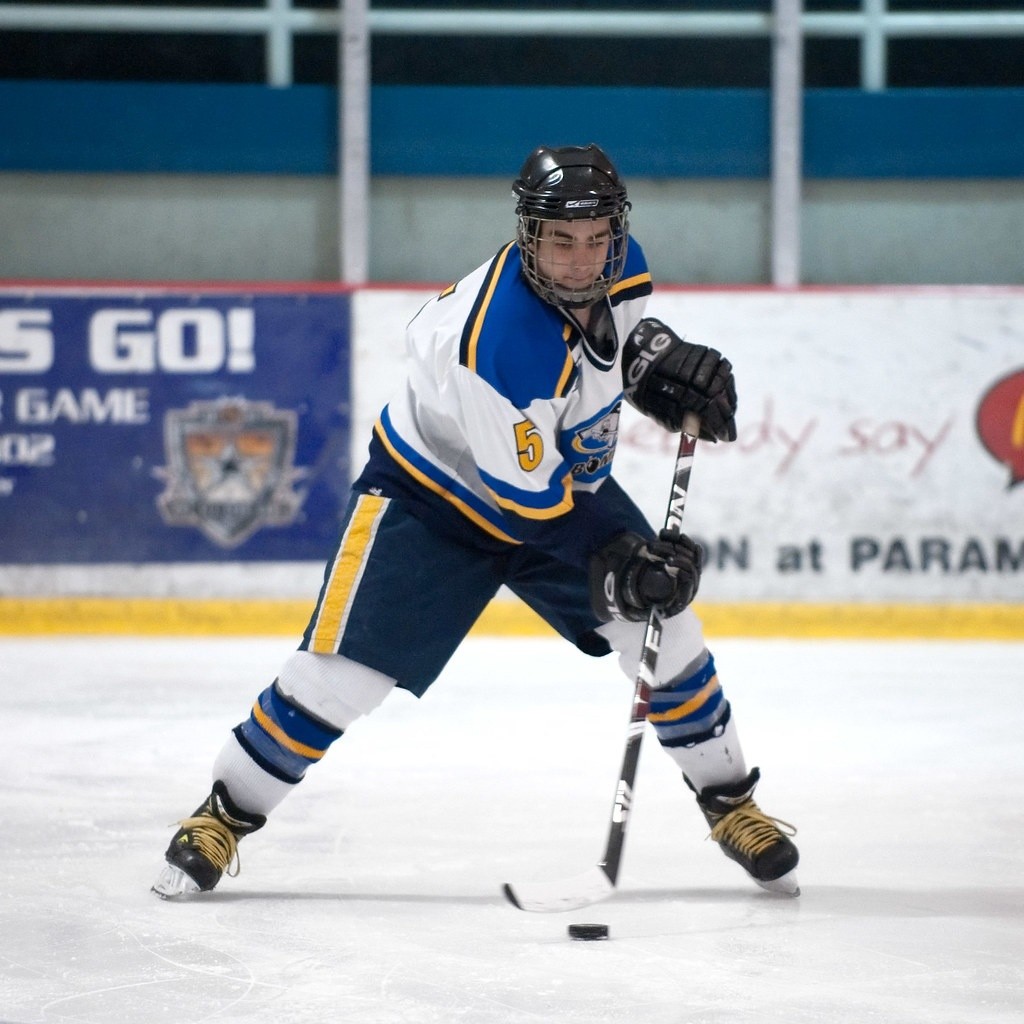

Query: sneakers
<box><xmin>682</xmin><ymin>767</ymin><xmax>801</xmax><ymax>897</ymax></box>
<box><xmin>148</xmin><ymin>780</ymin><xmax>267</xmax><ymax>900</ymax></box>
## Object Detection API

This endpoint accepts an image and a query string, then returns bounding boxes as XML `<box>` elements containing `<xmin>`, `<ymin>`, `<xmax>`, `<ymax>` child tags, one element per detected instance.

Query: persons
<box><xmin>149</xmin><ymin>144</ymin><xmax>803</xmax><ymax>901</ymax></box>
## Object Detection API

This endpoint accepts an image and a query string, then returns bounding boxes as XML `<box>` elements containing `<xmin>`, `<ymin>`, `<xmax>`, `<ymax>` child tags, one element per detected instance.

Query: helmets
<box><xmin>511</xmin><ymin>142</ymin><xmax>627</xmax><ymax>309</ymax></box>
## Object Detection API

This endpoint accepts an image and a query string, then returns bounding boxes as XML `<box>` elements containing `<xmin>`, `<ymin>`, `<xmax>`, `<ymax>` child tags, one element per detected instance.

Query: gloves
<box><xmin>622</xmin><ymin>317</ymin><xmax>737</xmax><ymax>443</ymax></box>
<box><xmin>588</xmin><ymin>523</ymin><xmax>702</xmax><ymax>622</ymax></box>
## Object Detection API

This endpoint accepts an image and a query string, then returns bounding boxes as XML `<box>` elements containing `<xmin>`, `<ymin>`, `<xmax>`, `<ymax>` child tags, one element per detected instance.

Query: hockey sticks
<box><xmin>503</xmin><ymin>416</ymin><xmax>702</xmax><ymax>912</ymax></box>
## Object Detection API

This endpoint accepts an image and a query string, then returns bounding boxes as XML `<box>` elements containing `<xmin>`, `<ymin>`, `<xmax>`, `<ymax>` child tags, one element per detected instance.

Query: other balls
<box><xmin>568</xmin><ymin>924</ymin><xmax>608</xmax><ymax>941</ymax></box>
